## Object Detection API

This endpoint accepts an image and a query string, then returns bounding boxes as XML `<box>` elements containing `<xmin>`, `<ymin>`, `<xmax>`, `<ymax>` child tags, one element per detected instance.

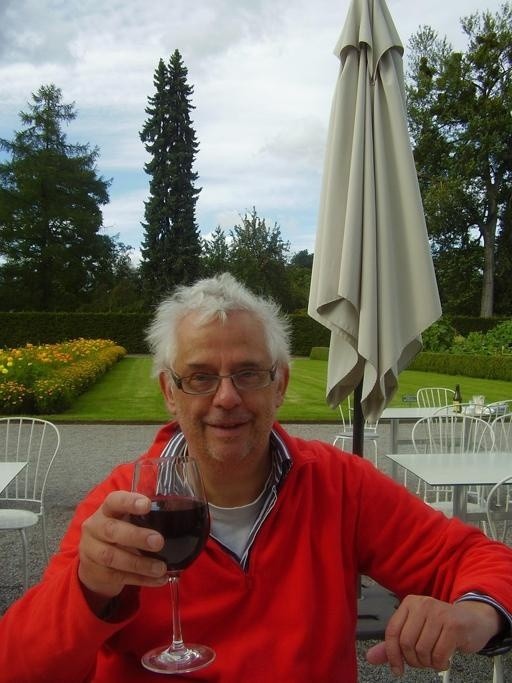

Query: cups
<box><xmin>466</xmin><ymin>395</ymin><xmax>506</xmax><ymax>415</ymax></box>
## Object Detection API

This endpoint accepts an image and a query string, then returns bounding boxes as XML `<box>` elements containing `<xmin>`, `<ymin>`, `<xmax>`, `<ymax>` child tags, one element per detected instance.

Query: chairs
<box><xmin>416</xmin><ymin>388</ymin><xmax>455</xmax><ymax>408</ymax></box>
<box><xmin>486</xmin><ymin>476</ymin><xmax>512</xmax><ymax>550</ymax></box>
<box><xmin>332</xmin><ymin>391</ymin><xmax>379</xmax><ymax>470</ymax></box>
<box><xmin>480</xmin><ymin>400</ymin><xmax>512</xmax><ymax>454</ymax></box>
<box><xmin>424</xmin><ymin>403</ymin><xmax>491</xmax><ymax>454</ymax></box>
<box><xmin>411</xmin><ymin>414</ymin><xmax>495</xmax><ymax>536</ymax></box>
<box><xmin>0</xmin><ymin>417</ymin><xmax>61</xmax><ymax>595</ymax></box>
<box><xmin>491</xmin><ymin>413</ymin><xmax>512</xmax><ymax>452</ymax></box>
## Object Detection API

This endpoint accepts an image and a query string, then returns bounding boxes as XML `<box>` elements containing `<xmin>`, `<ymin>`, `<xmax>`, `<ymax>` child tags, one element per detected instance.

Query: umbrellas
<box><xmin>302</xmin><ymin>0</ymin><xmax>446</xmax><ymax>460</ymax></box>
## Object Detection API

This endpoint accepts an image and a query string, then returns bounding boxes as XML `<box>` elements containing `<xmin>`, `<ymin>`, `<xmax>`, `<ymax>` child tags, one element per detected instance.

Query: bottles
<box><xmin>453</xmin><ymin>384</ymin><xmax>462</xmax><ymax>412</ymax></box>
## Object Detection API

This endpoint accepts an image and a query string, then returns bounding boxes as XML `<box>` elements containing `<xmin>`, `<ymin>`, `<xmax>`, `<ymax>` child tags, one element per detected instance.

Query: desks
<box><xmin>0</xmin><ymin>462</ymin><xmax>28</xmax><ymax>495</ymax></box>
<box><xmin>380</xmin><ymin>406</ymin><xmax>512</xmax><ymax>482</ymax></box>
<box><xmin>384</xmin><ymin>454</ymin><xmax>512</xmax><ymax>524</ymax></box>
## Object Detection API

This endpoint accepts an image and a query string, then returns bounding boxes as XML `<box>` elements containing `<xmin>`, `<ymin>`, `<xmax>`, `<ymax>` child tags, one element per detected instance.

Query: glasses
<box><xmin>164</xmin><ymin>361</ymin><xmax>277</xmax><ymax>395</ymax></box>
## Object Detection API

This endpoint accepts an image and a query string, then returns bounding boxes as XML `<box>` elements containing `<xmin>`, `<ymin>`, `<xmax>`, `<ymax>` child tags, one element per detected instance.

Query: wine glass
<box><xmin>130</xmin><ymin>457</ymin><xmax>217</xmax><ymax>675</ymax></box>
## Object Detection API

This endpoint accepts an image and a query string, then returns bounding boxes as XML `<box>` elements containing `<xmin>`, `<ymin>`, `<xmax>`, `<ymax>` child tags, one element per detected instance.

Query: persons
<box><xmin>1</xmin><ymin>267</ymin><xmax>512</xmax><ymax>682</ymax></box>
